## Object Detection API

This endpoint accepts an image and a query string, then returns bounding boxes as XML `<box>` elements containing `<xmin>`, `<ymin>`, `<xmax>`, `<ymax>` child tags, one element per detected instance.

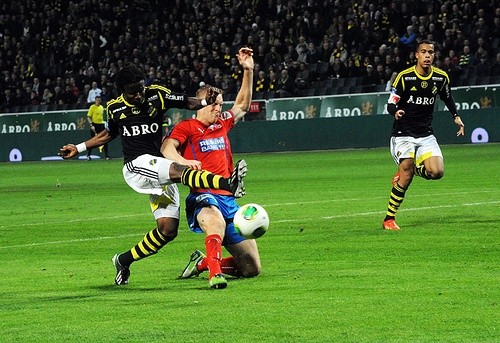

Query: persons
<box><xmin>86</xmin><ymin>96</ymin><xmax>111</xmax><ymax>161</ymax></box>
<box><xmin>0</xmin><ymin>0</ymin><xmax>500</xmax><ymax>113</ymax></box>
<box><xmin>161</xmin><ymin>47</ymin><xmax>262</xmax><ymax>288</ymax></box>
<box><xmin>383</xmin><ymin>40</ymin><xmax>465</xmax><ymax>231</ymax></box>
<box><xmin>60</xmin><ymin>66</ymin><xmax>248</xmax><ymax>287</ymax></box>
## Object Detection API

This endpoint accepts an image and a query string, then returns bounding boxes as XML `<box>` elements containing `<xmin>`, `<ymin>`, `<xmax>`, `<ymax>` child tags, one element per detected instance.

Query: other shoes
<box><xmin>86</xmin><ymin>155</ymin><xmax>91</xmax><ymax>160</ymax></box>
<box><xmin>103</xmin><ymin>155</ymin><xmax>110</xmax><ymax>160</ymax></box>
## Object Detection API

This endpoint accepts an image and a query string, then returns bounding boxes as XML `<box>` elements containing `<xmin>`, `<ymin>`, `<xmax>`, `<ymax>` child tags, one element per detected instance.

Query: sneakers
<box><xmin>392</xmin><ymin>171</ymin><xmax>401</xmax><ymax>187</ymax></box>
<box><xmin>229</xmin><ymin>157</ymin><xmax>248</xmax><ymax>198</ymax></box>
<box><xmin>179</xmin><ymin>249</ymin><xmax>206</xmax><ymax>278</ymax></box>
<box><xmin>208</xmin><ymin>274</ymin><xmax>227</xmax><ymax>289</ymax></box>
<box><xmin>111</xmin><ymin>253</ymin><xmax>131</xmax><ymax>286</ymax></box>
<box><xmin>382</xmin><ymin>218</ymin><xmax>401</xmax><ymax>232</ymax></box>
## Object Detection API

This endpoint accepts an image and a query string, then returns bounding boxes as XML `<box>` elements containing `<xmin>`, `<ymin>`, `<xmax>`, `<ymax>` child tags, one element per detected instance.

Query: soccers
<box><xmin>234</xmin><ymin>203</ymin><xmax>269</xmax><ymax>239</ymax></box>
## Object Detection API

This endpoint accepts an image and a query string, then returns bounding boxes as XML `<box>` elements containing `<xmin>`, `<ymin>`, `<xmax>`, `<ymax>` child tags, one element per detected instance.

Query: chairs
<box><xmin>307</xmin><ymin>62</ymin><xmax>500</xmax><ymax>98</ymax></box>
